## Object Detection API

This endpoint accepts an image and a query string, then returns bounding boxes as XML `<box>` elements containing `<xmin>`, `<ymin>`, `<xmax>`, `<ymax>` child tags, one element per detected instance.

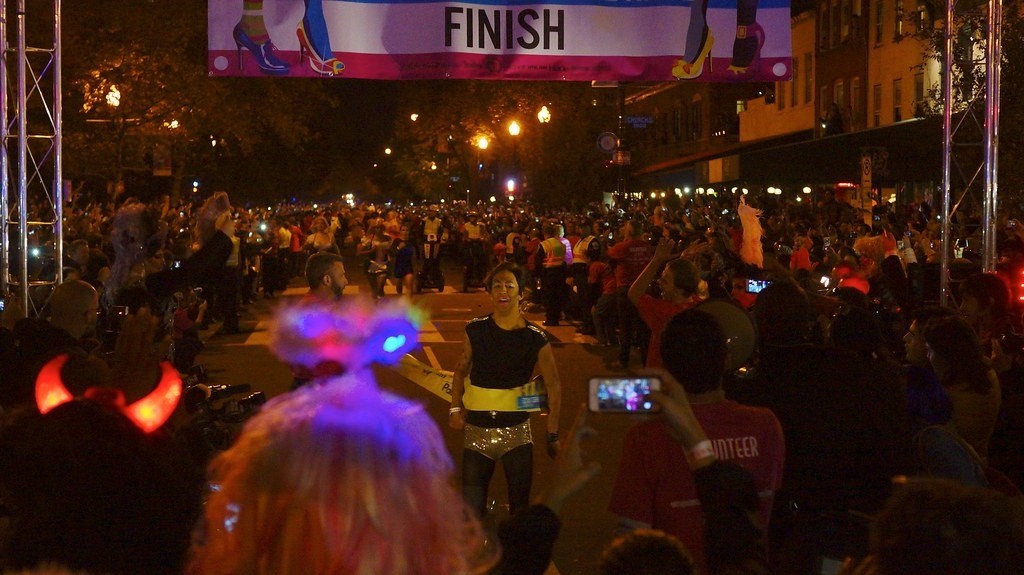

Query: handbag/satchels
<box><xmin>368</xmin><ymin>234</ymin><xmax>376</xmax><ymax>260</ymax></box>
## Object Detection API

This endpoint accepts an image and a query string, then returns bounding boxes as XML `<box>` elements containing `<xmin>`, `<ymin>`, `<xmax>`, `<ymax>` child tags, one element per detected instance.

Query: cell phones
<box><xmin>587</xmin><ymin>373</ymin><xmax>664</xmax><ymax>415</ymax></box>
<box><xmin>745</xmin><ymin>279</ymin><xmax>773</xmax><ymax>294</ymax></box>
<box><xmin>823</xmin><ymin>237</ymin><xmax>831</xmax><ymax>254</ymax></box>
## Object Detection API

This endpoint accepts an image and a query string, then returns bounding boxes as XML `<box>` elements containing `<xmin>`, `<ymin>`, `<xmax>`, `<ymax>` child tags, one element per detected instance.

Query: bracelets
<box><xmin>545</xmin><ymin>431</ymin><xmax>559</xmax><ymax>442</ymax></box>
<box><xmin>449</xmin><ymin>407</ymin><xmax>461</xmax><ymax>416</ymax></box>
<box><xmin>685</xmin><ymin>441</ymin><xmax>714</xmax><ymax>463</ymax></box>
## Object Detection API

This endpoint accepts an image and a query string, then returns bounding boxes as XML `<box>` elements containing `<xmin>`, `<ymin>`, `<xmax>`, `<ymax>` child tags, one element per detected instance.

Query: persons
<box><xmin>818</xmin><ymin>108</ymin><xmax>843</xmax><ymax>135</ymax></box>
<box><xmin>0</xmin><ymin>183</ymin><xmax>1024</xmax><ymax>575</ymax></box>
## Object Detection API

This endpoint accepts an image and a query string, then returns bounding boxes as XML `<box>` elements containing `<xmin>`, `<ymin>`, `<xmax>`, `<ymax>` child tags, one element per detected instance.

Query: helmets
<box><xmin>467</xmin><ymin>211</ymin><xmax>479</xmax><ymax>218</ymax></box>
<box><xmin>427</xmin><ymin>205</ymin><xmax>441</xmax><ymax>213</ymax></box>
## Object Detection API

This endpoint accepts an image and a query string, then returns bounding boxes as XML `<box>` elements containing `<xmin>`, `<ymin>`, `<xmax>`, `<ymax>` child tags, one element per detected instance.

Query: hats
<box><xmin>579</xmin><ymin>220</ymin><xmax>592</xmax><ymax>227</ymax></box>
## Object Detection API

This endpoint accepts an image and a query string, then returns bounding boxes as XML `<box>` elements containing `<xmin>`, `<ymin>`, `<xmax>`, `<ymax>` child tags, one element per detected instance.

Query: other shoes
<box><xmin>543</xmin><ymin>319</ymin><xmax>560</xmax><ymax>327</ymax></box>
<box><xmin>212</xmin><ymin>326</ymin><xmax>240</xmax><ymax>338</ymax></box>
